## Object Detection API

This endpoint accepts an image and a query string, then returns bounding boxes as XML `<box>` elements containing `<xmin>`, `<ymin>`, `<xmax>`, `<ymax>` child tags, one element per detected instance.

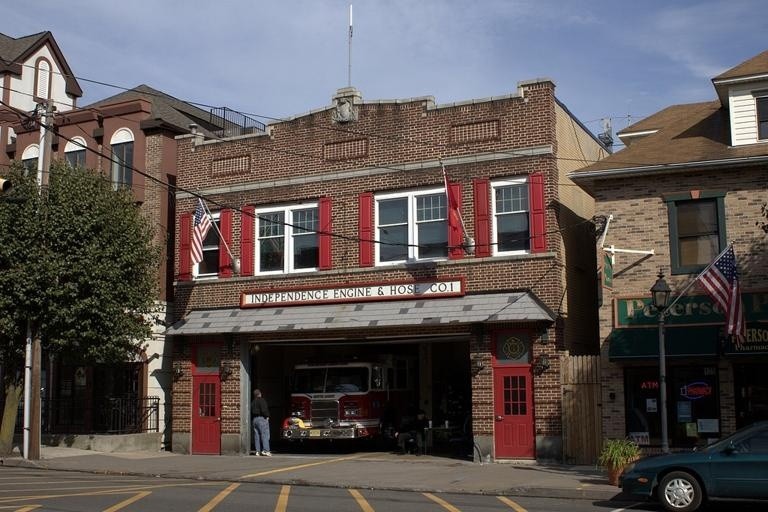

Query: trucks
<box><xmin>281</xmin><ymin>357</ymin><xmax>416</xmax><ymax>446</ymax></box>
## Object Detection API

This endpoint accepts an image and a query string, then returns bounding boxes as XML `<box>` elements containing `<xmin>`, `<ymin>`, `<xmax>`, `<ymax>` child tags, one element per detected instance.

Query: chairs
<box><xmin>406</xmin><ymin>420</ymin><xmax>432</xmax><ymax>454</ymax></box>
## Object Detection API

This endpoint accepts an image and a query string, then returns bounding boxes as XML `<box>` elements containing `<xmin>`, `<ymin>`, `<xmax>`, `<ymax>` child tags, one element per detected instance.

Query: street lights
<box><xmin>648</xmin><ymin>265</ymin><xmax>675</xmax><ymax>455</ymax></box>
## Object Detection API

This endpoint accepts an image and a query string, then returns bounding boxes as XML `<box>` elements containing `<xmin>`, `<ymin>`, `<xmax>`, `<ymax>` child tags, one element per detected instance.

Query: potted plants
<box><xmin>596</xmin><ymin>437</ymin><xmax>639</xmax><ymax>486</ymax></box>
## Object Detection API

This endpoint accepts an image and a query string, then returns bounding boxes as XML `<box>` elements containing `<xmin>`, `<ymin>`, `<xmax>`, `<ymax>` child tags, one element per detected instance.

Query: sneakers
<box><xmin>255</xmin><ymin>451</ymin><xmax>272</xmax><ymax>457</ymax></box>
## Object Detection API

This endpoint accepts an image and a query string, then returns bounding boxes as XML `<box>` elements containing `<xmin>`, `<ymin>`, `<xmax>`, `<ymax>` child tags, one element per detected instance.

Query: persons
<box><xmin>251</xmin><ymin>388</ymin><xmax>273</xmax><ymax>456</ymax></box>
<box><xmin>394</xmin><ymin>409</ymin><xmax>428</xmax><ymax>456</ymax></box>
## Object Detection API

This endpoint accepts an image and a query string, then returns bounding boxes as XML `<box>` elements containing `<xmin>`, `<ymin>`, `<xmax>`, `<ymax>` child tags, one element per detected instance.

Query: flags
<box><xmin>190</xmin><ymin>199</ymin><xmax>212</xmax><ymax>264</ymax></box>
<box><xmin>695</xmin><ymin>244</ymin><xmax>747</xmax><ymax>339</ymax></box>
<box><xmin>445</xmin><ymin>171</ymin><xmax>458</xmax><ymax>232</ymax></box>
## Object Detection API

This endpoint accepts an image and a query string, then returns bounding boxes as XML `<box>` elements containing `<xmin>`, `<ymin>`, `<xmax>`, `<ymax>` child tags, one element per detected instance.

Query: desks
<box><xmin>424</xmin><ymin>427</ymin><xmax>457</xmax><ymax>455</ymax></box>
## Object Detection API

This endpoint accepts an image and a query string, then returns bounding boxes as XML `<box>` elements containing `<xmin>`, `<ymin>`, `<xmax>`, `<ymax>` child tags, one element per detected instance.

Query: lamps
<box><xmin>473</xmin><ymin>353</ymin><xmax>484</xmax><ymax>375</ymax></box>
<box><xmin>221</xmin><ymin>361</ymin><xmax>232</xmax><ymax>382</ymax></box>
<box><xmin>175</xmin><ymin>361</ymin><xmax>184</xmax><ymax>379</ymax></box>
<box><xmin>540</xmin><ymin>349</ymin><xmax>549</xmax><ymax>373</ymax></box>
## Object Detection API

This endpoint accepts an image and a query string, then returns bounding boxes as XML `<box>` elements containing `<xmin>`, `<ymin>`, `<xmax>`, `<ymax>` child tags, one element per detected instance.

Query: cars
<box><xmin>618</xmin><ymin>415</ymin><xmax>768</xmax><ymax>511</ymax></box>
